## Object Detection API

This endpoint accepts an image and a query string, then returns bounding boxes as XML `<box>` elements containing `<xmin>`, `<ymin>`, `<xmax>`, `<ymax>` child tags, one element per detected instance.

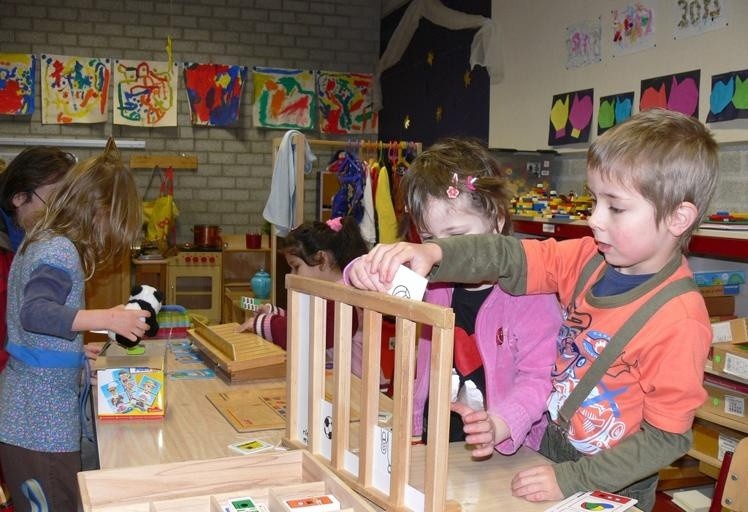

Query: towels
<box><xmin>262</xmin><ymin>129</ymin><xmax>317</xmax><ymax>239</ymax></box>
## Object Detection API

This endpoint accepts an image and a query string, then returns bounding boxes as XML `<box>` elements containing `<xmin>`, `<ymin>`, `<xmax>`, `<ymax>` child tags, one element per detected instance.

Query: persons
<box><xmin>1</xmin><ymin>140</ymin><xmax>77</xmax><ymax>372</ymax></box>
<box><xmin>316</xmin><ymin>136</ymin><xmax>566</xmax><ymax>458</ymax></box>
<box><xmin>235</xmin><ymin>216</ymin><xmax>391</xmax><ymax>397</ymax></box>
<box><xmin>0</xmin><ymin>155</ymin><xmax>154</xmax><ymax>512</ymax></box>
<box><xmin>361</xmin><ymin>105</ymin><xmax>721</xmax><ymax>512</ymax></box>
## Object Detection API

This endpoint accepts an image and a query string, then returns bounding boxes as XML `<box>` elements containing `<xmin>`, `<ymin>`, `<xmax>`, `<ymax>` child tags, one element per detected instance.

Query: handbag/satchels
<box><xmin>140</xmin><ymin>194</ymin><xmax>180</xmax><ymax>243</ymax></box>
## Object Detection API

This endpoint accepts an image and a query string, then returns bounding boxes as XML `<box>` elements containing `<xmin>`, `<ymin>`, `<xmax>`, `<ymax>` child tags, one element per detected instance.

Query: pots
<box><xmin>190</xmin><ymin>223</ymin><xmax>222</xmax><ymax>248</ymax></box>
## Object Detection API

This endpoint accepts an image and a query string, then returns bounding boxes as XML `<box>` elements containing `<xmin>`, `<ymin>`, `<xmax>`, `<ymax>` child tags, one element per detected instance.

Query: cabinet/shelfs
<box><xmin>164</xmin><ymin>232</ymin><xmax>273</xmax><ymax>324</ymax></box>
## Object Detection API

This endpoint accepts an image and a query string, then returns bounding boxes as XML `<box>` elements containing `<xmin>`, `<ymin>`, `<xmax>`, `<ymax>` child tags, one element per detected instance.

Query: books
<box><xmin>206</xmin><ymin>386</ymin><xmax>361</xmax><ymax>432</ymax></box>
<box><xmin>671</xmin><ymin>485</ymin><xmax>716</xmax><ymax>512</ymax></box>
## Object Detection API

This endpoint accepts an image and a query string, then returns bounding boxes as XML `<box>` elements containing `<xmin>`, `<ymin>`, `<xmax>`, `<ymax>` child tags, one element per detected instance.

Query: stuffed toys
<box><xmin>107</xmin><ymin>283</ymin><xmax>165</xmax><ymax>347</ymax></box>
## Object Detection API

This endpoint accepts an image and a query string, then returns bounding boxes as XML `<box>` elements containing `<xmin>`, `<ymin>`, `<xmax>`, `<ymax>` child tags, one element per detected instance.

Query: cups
<box><xmin>244</xmin><ymin>231</ymin><xmax>262</xmax><ymax>249</ymax></box>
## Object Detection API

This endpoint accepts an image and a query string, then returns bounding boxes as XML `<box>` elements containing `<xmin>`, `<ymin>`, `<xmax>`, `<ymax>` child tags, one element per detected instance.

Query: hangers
<box><xmin>326</xmin><ymin>138</ymin><xmax>418</xmax><ymax>182</ymax></box>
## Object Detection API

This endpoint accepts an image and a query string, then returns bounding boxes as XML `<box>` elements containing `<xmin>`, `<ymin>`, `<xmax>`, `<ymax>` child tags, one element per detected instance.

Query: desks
<box><xmin>86</xmin><ymin>338</ymin><xmax>643</xmax><ymax>511</ymax></box>
